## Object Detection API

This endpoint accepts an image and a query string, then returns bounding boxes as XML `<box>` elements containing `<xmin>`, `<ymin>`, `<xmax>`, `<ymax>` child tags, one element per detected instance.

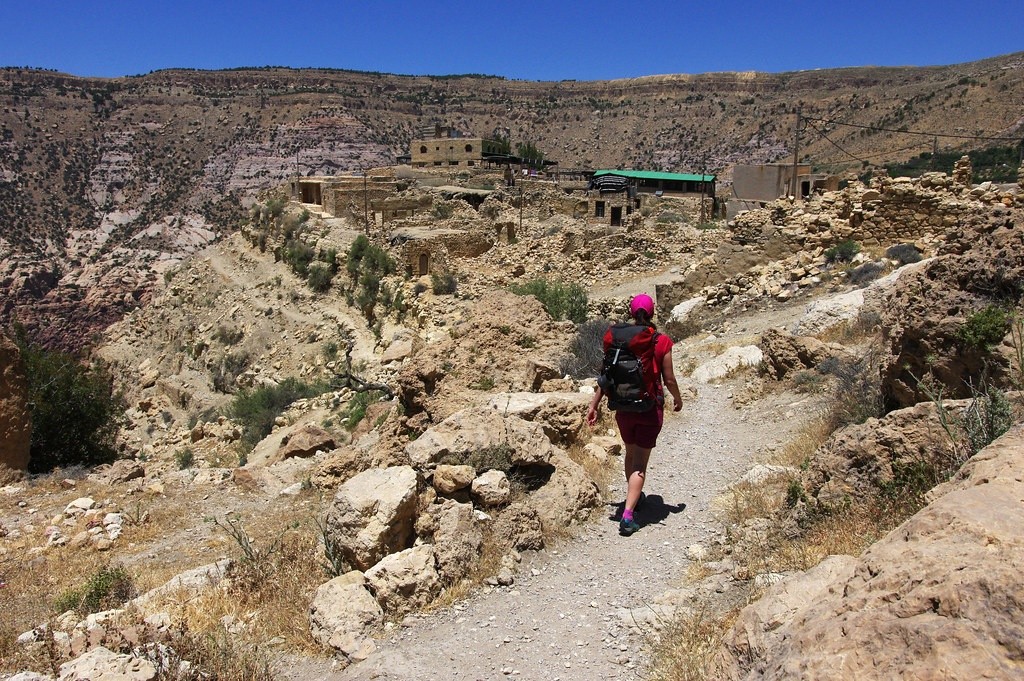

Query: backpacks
<box><xmin>597</xmin><ymin>323</ymin><xmax>661</xmax><ymax>411</ymax></box>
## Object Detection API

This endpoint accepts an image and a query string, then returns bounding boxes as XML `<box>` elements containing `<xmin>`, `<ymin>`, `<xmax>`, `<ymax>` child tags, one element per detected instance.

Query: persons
<box><xmin>587</xmin><ymin>294</ymin><xmax>683</xmax><ymax>536</ymax></box>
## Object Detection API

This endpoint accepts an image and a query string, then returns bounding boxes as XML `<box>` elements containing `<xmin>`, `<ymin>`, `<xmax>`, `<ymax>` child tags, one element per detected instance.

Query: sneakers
<box><xmin>620</xmin><ymin>518</ymin><xmax>639</xmax><ymax>534</ymax></box>
<box><xmin>635</xmin><ymin>492</ymin><xmax>645</xmax><ymax>511</ymax></box>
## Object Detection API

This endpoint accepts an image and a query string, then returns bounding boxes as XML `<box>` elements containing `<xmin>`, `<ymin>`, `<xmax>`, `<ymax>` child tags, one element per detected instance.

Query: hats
<box><xmin>630</xmin><ymin>294</ymin><xmax>654</xmax><ymax>318</ymax></box>
